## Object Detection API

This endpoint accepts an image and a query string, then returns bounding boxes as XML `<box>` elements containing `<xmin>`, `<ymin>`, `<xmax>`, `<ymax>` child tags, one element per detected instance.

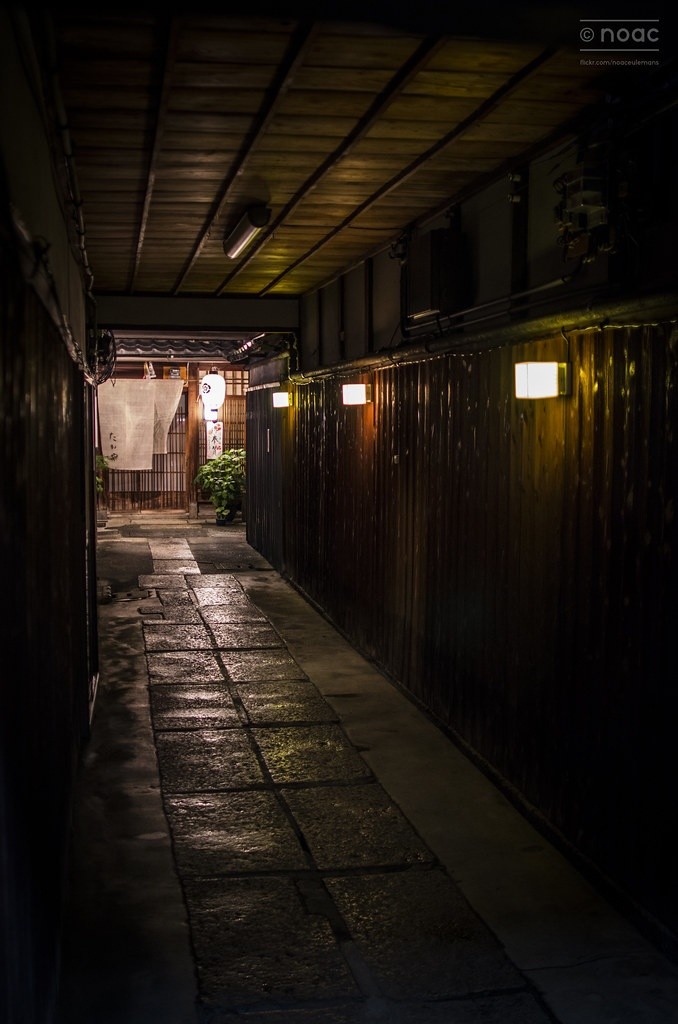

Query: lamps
<box><xmin>342</xmin><ymin>384</ymin><xmax>372</xmax><ymax>405</ymax></box>
<box><xmin>515</xmin><ymin>361</ymin><xmax>570</xmax><ymax>399</ymax></box>
<box><xmin>272</xmin><ymin>392</ymin><xmax>292</xmax><ymax>408</ymax></box>
<box><xmin>223</xmin><ymin>206</ymin><xmax>273</xmax><ymax>259</ymax></box>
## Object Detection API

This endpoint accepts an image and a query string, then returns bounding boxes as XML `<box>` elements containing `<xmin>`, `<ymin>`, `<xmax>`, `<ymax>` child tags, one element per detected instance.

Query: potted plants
<box><xmin>196</xmin><ymin>448</ymin><xmax>246</xmax><ymax>526</ymax></box>
